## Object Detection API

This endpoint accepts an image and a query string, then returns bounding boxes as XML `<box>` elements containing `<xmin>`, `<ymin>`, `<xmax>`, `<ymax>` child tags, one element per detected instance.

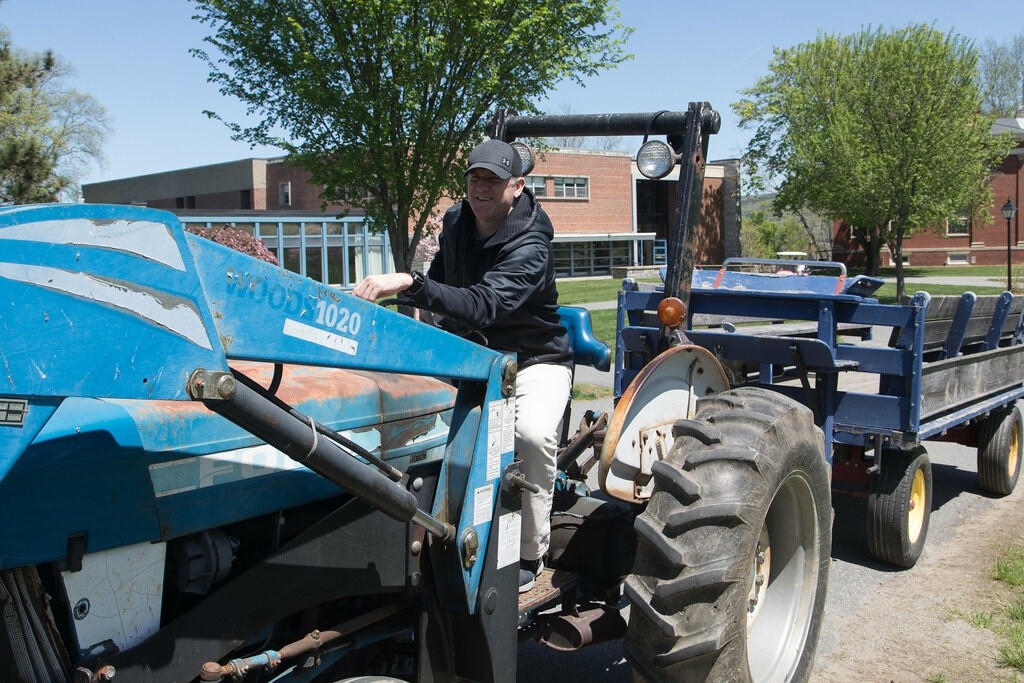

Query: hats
<box><xmin>463</xmin><ymin>139</ymin><xmax>523</xmax><ymax>180</ymax></box>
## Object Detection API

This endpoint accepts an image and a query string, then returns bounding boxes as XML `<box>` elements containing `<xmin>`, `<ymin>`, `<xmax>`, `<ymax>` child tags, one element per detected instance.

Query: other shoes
<box><xmin>518</xmin><ymin>557</ymin><xmax>545</xmax><ymax>593</ymax></box>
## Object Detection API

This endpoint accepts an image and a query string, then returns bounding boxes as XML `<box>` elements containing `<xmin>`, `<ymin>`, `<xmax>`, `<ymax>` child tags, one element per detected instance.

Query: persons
<box><xmin>353</xmin><ymin>139</ymin><xmax>575</xmax><ymax>592</ymax></box>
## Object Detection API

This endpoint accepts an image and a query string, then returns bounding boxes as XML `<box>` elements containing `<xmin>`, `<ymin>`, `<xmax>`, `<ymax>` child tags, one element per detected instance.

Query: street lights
<box><xmin>1000</xmin><ymin>195</ymin><xmax>1018</xmax><ymax>291</ymax></box>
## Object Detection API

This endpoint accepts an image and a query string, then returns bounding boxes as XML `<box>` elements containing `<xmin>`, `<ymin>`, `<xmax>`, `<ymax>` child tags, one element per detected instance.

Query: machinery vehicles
<box><xmin>1</xmin><ymin>102</ymin><xmax>1024</xmax><ymax>683</ymax></box>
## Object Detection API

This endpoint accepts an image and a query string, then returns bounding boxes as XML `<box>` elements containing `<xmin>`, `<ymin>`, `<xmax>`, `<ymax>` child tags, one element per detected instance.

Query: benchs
<box><xmin>694</xmin><ymin>323</ymin><xmax>872</xmax><ymax>336</ymax></box>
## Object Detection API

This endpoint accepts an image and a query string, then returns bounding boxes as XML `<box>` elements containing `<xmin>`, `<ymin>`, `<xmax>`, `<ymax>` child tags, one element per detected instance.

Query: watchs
<box><xmin>401</xmin><ymin>271</ymin><xmax>425</xmax><ymax>297</ymax></box>
